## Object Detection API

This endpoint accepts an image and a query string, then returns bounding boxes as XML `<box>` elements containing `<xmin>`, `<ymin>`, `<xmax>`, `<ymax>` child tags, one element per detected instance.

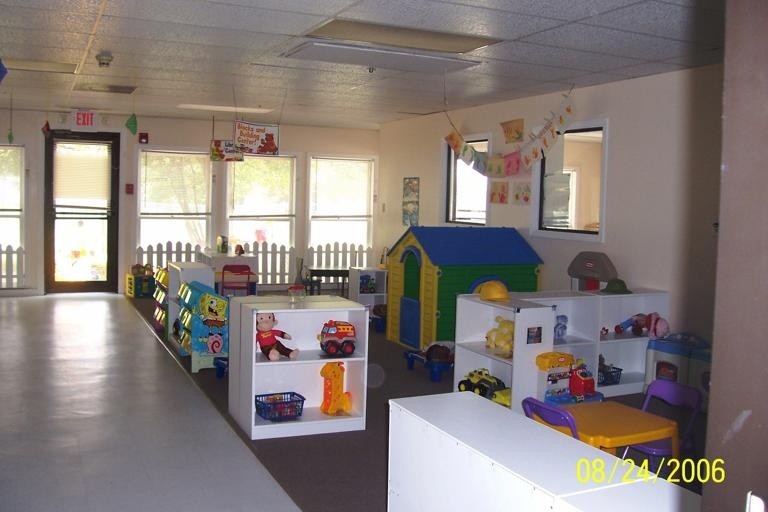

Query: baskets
<box><xmin>255</xmin><ymin>392</ymin><xmax>305</xmax><ymax>420</ymax></box>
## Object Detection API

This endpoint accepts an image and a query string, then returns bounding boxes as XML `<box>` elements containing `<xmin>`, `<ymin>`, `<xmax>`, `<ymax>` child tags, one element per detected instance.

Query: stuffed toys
<box><xmin>485</xmin><ymin>315</ymin><xmax>515</xmax><ymax>353</ymax></box>
<box><xmin>256</xmin><ymin>313</ymin><xmax>300</xmax><ymax>361</ymax></box>
<box><xmin>614</xmin><ymin>311</ymin><xmax>670</xmax><ymax>340</ymax></box>
